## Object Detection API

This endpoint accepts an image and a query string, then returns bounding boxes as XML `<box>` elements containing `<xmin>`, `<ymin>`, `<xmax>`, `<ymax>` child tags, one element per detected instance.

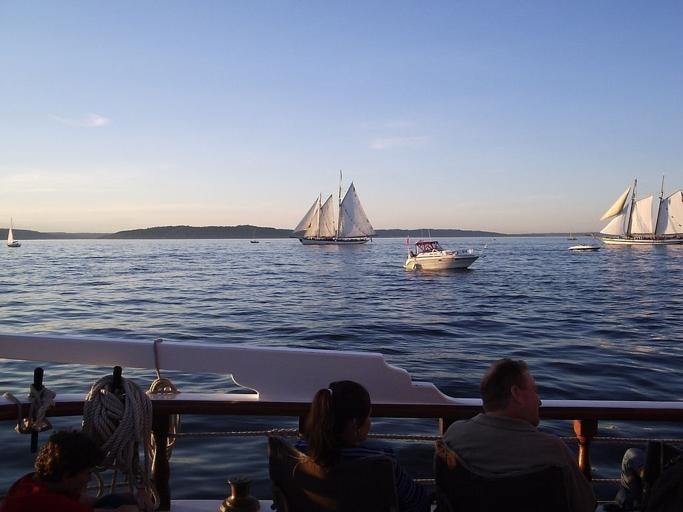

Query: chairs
<box><xmin>267</xmin><ymin>433</ymin><xmax>396</xmax><ymax>511</ymax></box>
<box><xmin>431</xmin><ymin>435</ymin><xmax>596</xmax><ymax>511</ymax></box>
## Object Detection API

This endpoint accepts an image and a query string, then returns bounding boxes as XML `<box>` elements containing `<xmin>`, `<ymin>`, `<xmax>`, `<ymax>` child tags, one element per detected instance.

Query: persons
<box><xmin>433</xmin><ymin>358</ymin><xmax>598</xmax><ymax>511</ymax></box>
<box><xmin>611</xmin><ymin>446</ymin><xmax>650</xmax><ymax>511</ymax></box>
<box><xmin>1</xmin><ymin>423</ymin><xmax>155</xmax><ymax>512</ymax></box>
<box><xmin>273</xmin><ymin>379</ymin><xmax>432</xmax><ymax>512</ymax></box>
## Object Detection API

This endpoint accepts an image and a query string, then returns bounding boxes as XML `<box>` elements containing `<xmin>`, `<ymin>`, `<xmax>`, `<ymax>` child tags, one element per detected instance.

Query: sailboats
<box><xmin>591</xmin><ymin>173</ymin><xmax>682</xmax><ymax>244</ymax></box>
<box><xmin>567</xmin><ymin>232</ymin><xmax>576</xmax><ymax>240</ymax></box>
<box><xmin>6</xmin><ymin>217</ymin><xmax>20</xmax><ymax>248</ymax></box>
<box><xmin>292</xmin><ymin>170</ymin><xmax>378</xmax><ymax>245</ymax></box>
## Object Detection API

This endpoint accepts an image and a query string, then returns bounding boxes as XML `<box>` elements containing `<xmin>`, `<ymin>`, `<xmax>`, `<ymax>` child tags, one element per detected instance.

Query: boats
<box><xmin>400</xmin><ymin>226</ymin><xmax>478</xmax><ymax>272</ymax></box>
<box><xmin>250</xmin><ymin>240</ymin><xmax>259</xmax><ymax>244</ymax></box>
<box><xmin>568</xmin><ymin>244</ymin><xmax>600</xmax><ymax>249</ymax></box>
<box><xmin>569</xmin><ymin>249</ymin><xmax>600</xmax><ymax>253</ymax></box>
